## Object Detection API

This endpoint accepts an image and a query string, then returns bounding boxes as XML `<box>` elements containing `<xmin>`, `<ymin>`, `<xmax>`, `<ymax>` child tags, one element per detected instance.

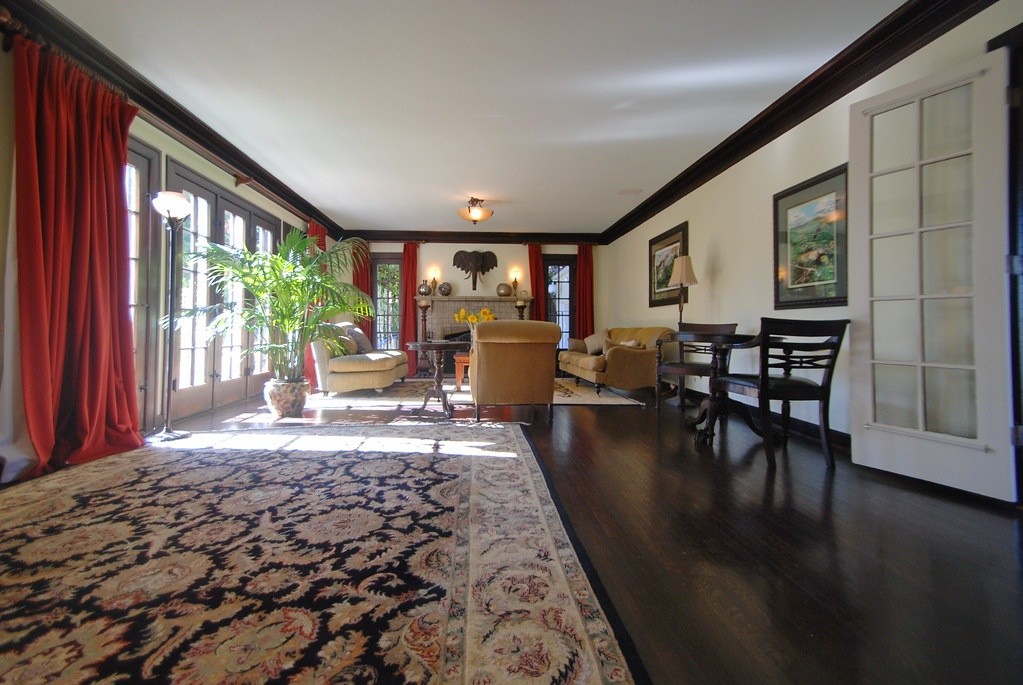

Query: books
<box><xmin>427</xmin><ymin>340</ymin><xmax>449</xmax><ymax>343</ymax></box>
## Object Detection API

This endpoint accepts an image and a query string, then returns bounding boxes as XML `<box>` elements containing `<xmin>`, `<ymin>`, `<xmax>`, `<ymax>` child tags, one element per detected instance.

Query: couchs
<box><xmin>310</xmin><ymin>321</ymin><xmax>409</xmax><ymax>397</ymax></box>
<box><xmin>557</xmin><ymin>327</ymin><xmax>676</xmax><ymax>397</ymax></box>
<box><xmin>468</xmin><ymin>319</ymin><xmax>561</xmax><ymax>423</ymax></box>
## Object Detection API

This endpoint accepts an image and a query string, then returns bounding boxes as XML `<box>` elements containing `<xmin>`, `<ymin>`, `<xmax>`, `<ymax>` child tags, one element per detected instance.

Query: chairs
<box><xmin>656</xmin><ymin>322</ymin><xmax>738</xmax><ymax>430</ymax></box>
<box><xmin>706</xmin><ymin>316</ymin><xmax>852</xmax><ymax>474</ymax></box>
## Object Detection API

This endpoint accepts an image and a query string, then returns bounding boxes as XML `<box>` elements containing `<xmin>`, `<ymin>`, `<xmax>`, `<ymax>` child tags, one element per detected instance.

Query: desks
<box><xmin>406</xmin><ymin>341</ymin><xmax>471</xmax><ymax>418</ymax></box>
<box><xmin>671</xmin><ymin>333</ymin><xmax>784</xmax><ymax>450</ymax></box>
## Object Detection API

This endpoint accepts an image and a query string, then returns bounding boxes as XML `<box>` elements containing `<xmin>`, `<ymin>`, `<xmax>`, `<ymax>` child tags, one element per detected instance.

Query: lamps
<box><xmin>664</xmin><ymin>256</ymin><xmax>699</xmax><ymax>408</ymax></box>
<box><xmin>510</xmin><ymin>268</ymin><xmax>521</xmax><ymax>296</ymax></box>
<box><xmin>457</xmin><ymin>197</ymin><xmax>494</xmax><ymax>225</ymax></box>
<box><xmin>144</xmin><ymin>191</ymin><xmax>195</xmax><ymax>442</ymax></box>
<box><xmin>428</xmin><ymin>267</ymin><xmax>440</xmax><ymax>296</ymax></box>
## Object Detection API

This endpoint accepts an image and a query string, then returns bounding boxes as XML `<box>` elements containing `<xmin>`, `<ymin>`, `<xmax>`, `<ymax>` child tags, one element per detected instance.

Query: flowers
<box><xmin>453</xmin><ymin>306</ymin><xmax>498</xmax><ymax>330</ymax></box>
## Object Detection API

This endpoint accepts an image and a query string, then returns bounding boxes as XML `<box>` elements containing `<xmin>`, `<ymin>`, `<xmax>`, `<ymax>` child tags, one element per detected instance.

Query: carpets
<box><xmin>0</xmin><ymin>421</ymin><xmax>653</xmax><ymax>685</ymax></box>
<box><xmin>306</xmin><ymin>378</ymin><xmax>646</xmax><ymax>405</ymax></box>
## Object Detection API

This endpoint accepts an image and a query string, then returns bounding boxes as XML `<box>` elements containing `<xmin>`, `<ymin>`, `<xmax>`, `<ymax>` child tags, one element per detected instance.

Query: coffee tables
<box><xmin>453</xmin><ymin>351</ymin><xmax>470</xmax><ymax>392</ymax></box>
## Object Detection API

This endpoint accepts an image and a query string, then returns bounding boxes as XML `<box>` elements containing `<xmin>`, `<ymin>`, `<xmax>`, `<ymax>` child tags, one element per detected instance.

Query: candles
<box><xmin>516</xmin><ymin>301</ymin><xmax>524</xmax><ymax>306</ymax></box>
<box><xmin>418</xmin><ymin>300</ymin><xmax>427</xmax><ymax>306</ymax></box>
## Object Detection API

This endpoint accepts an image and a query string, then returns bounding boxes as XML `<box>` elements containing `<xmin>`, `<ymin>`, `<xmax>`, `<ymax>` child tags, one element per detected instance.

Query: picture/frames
<box><xmin>772</xmin><ymin>160</ymin><xmax>849</xmax><ymax>310</ymax></box>
<box><xmin>648</xmin><ymin>220</ymin><xmax>689</xmax><ymax>308</ymax></box>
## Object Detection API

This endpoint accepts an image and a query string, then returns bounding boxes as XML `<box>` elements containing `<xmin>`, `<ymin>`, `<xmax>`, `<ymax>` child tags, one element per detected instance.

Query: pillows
<box><xmin>346</xmin><ymin>327</ymin><xmax>372</xmax><ymax>354</ymax></box>
<box><xmin>600</xmin><ymin>339</ymin><xmax>646</xmax><ymax>357</ymax></box>
<box><xmin>338</xmin><ymin>334</ymin><xmax>359</xmax><ymax>355</ymax></box>
<box><xmin>584</xmin><ymin>327</ymin><xmax>611</xmax><ymax>355</ymax></box>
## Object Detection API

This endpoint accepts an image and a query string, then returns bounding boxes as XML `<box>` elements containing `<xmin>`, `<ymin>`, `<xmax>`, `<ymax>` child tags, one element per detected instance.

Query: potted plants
<box><xmin>157</xmin><ymin>218</ymin><xmax>377</xmax><ymax>419</ymax></box>
